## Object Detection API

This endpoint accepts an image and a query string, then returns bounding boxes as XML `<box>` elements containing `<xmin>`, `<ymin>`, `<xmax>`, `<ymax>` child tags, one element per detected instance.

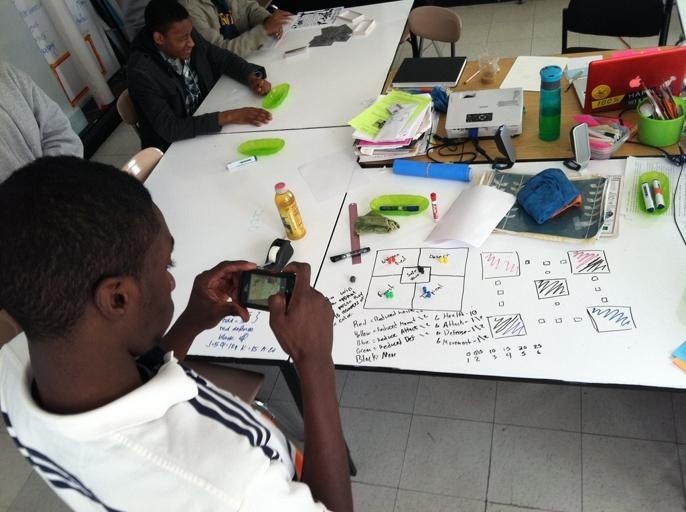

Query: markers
<box><xmin>641</xmin><ymin>182</ymin><xmax>654</xmax><ymax>212</ymax></box>
<box><xmin>226</xmin><ymin>155</ymin><xmax>258</xmax><ymax>170</ymax></box>
<box><xmin>652</xmin><ymin>179</ymin><xmax>665</xmax><ymax>209</ymax></box>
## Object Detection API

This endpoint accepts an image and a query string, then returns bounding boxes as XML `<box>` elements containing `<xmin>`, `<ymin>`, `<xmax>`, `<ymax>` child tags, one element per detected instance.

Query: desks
<box><xmin>286</xmin><ymin>138</ymin><xmax>686</xmax><ymax>391</ymax></box>
<box><xmin>130</xmin><ymin>123</ymin><xmax>373</xmax><ymax>478</ymax></box>
<box><xmin>354</xmin><ymin>47</ymin><xmax>686</xmax><ymax>170</ymax></box>
<box><xmin>187</xmin><ymin>1</ymin><xmax>422</xmax><ymax>137</ymax></box>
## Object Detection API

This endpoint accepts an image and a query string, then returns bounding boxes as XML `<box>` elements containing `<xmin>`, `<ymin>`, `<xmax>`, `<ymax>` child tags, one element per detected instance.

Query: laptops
<box><xmin>572</xmin><ymin>45</ymin><xmax>686</xmax><ymax>114</ymax></box>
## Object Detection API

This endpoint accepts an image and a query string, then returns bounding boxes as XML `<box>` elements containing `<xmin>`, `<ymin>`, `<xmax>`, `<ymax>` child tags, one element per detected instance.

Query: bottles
<box><xmin>273</xmin><ymin>182</ymin><xmax>306</xmax><ymax>241</ymax></box>
<box><xmin>538</xmin><ymin>64</ymin><xmax>562</xmax><ymax>142</ymax></box>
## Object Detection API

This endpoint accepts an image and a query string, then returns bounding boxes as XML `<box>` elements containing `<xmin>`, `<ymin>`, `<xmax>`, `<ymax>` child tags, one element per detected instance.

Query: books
<box><xmin>391</xmin><ymin>57</ymin><xmax>468</xmax><ymax>87</ymax></box>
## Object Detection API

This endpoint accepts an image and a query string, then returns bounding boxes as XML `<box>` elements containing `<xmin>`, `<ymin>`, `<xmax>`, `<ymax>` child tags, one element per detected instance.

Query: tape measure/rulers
<box><xmin>349</xmin><ymin>203</ymin><xmax>361</xmax><ymax>265</ymax></box>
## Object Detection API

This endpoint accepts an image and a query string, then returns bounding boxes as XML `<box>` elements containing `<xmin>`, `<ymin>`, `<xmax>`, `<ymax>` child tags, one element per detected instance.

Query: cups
<box><xmin>477</xmin><ymin>52</ymin><xmax>500</xmax><ymax>85</ymax></box>
<box><xmin>637</xmin><ymin>94</ymin><xmax>686</xmax><ymax>148</ymax></box>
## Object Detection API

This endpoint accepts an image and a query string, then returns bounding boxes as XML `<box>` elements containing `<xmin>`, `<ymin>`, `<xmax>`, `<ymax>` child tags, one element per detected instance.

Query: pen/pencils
<box><xmin>640</xmin><ymin>78</ymin><xmax>680</xmax><ymax>121</ymax></box>
<box><xmin>330</xmin><ymin>247</ymin><xmax>370</xmax><ymax>263</ymax></box>
<box><xmin>431</xmin><ymin>193</ymin><xmax>439</xmax><ymax>222</ymax></box>
<box><xmin>379</xmin><ymin>205</ymin><xmax>419</xmax><ymax>212</ymax></box>
<box><xmin>564</xmin><ymin>70</ymin><xmax>583</xmax><ymax>93</ymax></box>
<box><xmin>285</xmin><ymin>46</ymin><xmax>307</xmax><ymax>54</ymax></box>
<box><xmin>464</xmin><ymin>61</ymin><xmax>491</xmax><ymax>84</ymax></box>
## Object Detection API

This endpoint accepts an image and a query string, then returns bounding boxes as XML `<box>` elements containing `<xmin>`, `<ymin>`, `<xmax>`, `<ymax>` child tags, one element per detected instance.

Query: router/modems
<box><xmin>445</xmin><ymin>87</ymin><xmax>523</xmax><ymax>140</ymax></box>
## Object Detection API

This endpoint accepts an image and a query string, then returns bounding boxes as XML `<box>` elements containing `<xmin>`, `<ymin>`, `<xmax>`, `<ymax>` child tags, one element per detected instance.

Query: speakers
<box><xmin>563</xmin><ymin>122</ymin><xmax>591</xmax><ymax>170</ymax></box>
<box><xmin>492</xmin><ymin>125</ymin><xmax>516</xmax><ymax>170</ymax></box>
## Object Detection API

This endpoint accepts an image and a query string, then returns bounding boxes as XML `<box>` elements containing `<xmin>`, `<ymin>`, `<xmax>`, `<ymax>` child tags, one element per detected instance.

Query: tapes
<box><xmin>259</xmin><ymin>245</ymin><xmax>281</xmax><ymax>267</ymax></box>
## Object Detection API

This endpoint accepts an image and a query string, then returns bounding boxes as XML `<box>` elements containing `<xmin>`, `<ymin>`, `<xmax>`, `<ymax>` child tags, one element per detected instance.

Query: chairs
<box><xmin>0</xmin><ymin>304</ymin><xmax>307</xmax><ymax>448</ymax></box>
<box><xmin>115</xmin><ymin>88</ymin><xmax>144</xmax><ymax>143</ymax></box>
<box><xmin>559</xmin><ymin>0</ymin><xmax>676</xmax><ymax>55</ymax></box>
<box><xmin>119</xmin><ymin>146</ymin><xmax>163</xmax><ymax>186</ymax></box>
<box><xmin>407</xmin><ymin>5</ymin><xmax>464</xmax><ymax>59</ymax></box>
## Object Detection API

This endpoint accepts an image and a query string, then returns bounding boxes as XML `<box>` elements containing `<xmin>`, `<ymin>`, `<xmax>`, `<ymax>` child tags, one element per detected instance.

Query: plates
<box><xmin>638</xmin><ymin>171</ymin><xmax>670</xmax><ymax>216</ymax></box>
<box><xmin>237</xmin><ymin>138</ymin><xmax>287</xmax><ymax>158</ymax></box>
<box><xmin>261</xmin><ymin>82</ymin><xmax>290</xmax><ymax>110</ymax></box>
<box><xmin>368</xmin><ymin>193</ymin><xmax>430</xmax><ymax>217</ymax></box>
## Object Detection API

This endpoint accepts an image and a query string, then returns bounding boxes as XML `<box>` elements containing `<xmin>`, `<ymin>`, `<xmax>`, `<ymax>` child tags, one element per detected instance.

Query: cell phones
<box><xmin>238</xmin><ymin>268</ymin><xmax>295</xmax><ymax>313</ymax></box>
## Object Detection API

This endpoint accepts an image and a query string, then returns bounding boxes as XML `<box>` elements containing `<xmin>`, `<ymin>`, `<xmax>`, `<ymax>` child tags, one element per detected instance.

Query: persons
<box><xmin>126</xmin><ymin>0</ymin><xmax>273</xmax><ymax>153</ymax></box>
<box><xmin>0</xmin><ymin>59</ymin><xmax>85</xmax><ymax>184</ymax></box>
<box><xmin>0</xmin><ymin>156</ymin><xmax>354</xmax><ymax>512</ymax></box>
<box><xmin>179</xmin><ymin>0</ymin><xmax>294</xmax><ymax>59</ymax></box>
